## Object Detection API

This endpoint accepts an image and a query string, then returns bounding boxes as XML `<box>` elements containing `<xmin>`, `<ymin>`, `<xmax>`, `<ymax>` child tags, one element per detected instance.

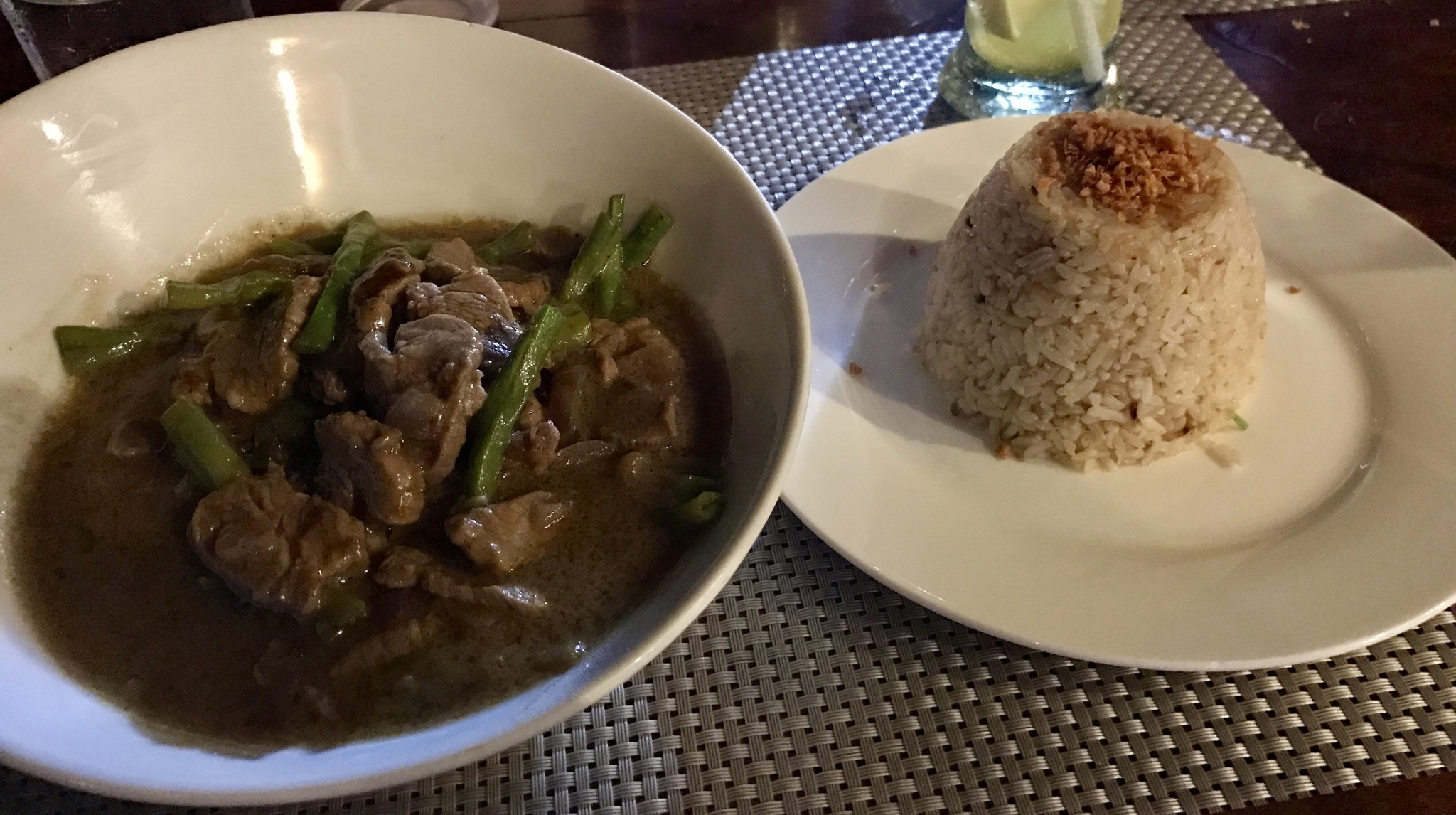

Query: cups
<box><xmin>935</xmin><ymin>1</ymin><xmax>1128</xmax><ymax>119</ymax></box>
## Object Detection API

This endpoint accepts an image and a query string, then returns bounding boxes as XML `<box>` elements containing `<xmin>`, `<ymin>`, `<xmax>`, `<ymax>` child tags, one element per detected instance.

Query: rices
<box><xmin>909</xmin><ymin>103</ymin><xmax>1269</xmax><ymax>482</ymax></box>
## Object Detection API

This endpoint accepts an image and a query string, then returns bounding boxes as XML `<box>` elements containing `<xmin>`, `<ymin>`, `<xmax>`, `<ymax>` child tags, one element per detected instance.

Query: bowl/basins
<box><xmin>0</xmin><ymin>12</ymin><xmax>813</xmax><ymax>806</ymax></box>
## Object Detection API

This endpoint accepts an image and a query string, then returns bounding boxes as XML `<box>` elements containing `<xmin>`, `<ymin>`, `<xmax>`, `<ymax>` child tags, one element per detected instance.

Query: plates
<box><xmin>780</xmin><ymin>113</ymin><xmax>1455</xmax><ymax>672</ymax></box>
